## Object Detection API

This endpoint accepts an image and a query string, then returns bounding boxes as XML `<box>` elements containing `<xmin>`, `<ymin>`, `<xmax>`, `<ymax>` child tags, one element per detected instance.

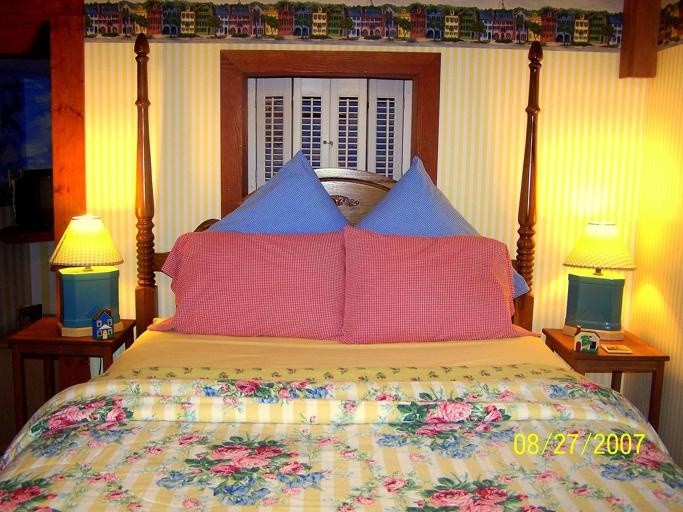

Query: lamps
<box><xmin>47</xmin><ymin>214</ymin><xmax>125</xmax><ymax>339</ymax></box>
<box><xmin>562</xmin><ymin>222</ymin><xmax>637</xmax><ymax>340</ymax></box>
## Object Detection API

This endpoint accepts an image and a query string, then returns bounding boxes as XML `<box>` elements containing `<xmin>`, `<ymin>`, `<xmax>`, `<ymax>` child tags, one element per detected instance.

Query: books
<box><xmin>600</xmin><ymin>344</ymin><xmax>632</xmax><ymax>353</ymax></box>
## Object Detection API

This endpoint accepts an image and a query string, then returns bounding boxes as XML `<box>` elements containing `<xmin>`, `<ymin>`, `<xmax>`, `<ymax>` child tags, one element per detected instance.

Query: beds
<box><xmin>0</xmin><ymin>32</ymin><xmax>683</xmax><ymax>512</ymax></box>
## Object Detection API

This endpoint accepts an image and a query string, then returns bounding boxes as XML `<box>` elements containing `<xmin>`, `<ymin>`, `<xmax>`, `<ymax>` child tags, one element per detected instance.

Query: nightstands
<box><xmin>542</xmin><ymin>329</ymin><xmax>670</xmax><ymax>434</ymax></box>
<box><xmin>8</xmin><ymin>317</ymin><xmax>139</xmax><ymax>431</ymax></box>
